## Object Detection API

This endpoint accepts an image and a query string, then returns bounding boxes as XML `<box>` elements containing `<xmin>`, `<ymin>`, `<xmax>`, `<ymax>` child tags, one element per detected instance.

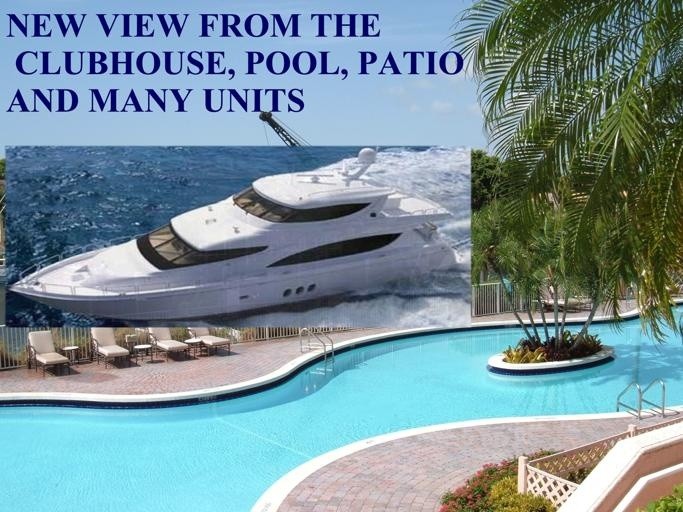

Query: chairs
<box><xmin>528</xmin><ymin>275</ymin><xmax>604</xmax><ymax>313</ymax></box>
<box><xmin>22</xmin><ymin>327</ymin><xmax>235</xmax><ymax>376</ymax></box>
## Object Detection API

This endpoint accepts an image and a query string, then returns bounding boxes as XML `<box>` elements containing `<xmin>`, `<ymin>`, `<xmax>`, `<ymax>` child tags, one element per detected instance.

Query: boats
<box><xmin>9</xmin><ymin>147</ymin><xmax>470</xmax><ymax>322</ymax></box>
